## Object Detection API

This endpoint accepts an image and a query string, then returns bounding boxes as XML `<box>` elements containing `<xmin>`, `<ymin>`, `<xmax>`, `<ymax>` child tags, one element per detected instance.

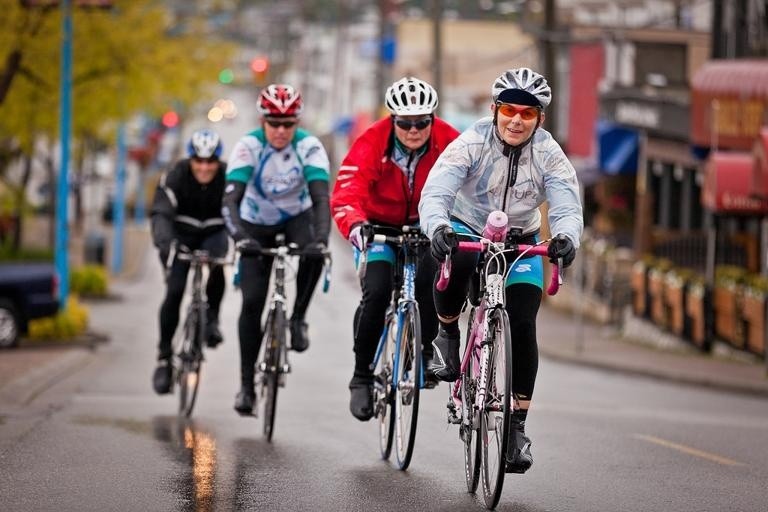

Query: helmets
<box><xmin>491</xmin><ymin>67</ymin><xmax>551</xmax><ymax>107</ymax></box>
<box><xmin>384</xmin><ymin>76</ymin><xmax>439</xmax><ymax>117</ymax></box>
<box><xmin>187</xmin><ymin>130</ymin><xmax>223</xmax><ymax>159</ymax></box>
<box><xmin>255</xmin><ymin>84</ymin><xmax>302</xmax><ymax>117</ymax></box>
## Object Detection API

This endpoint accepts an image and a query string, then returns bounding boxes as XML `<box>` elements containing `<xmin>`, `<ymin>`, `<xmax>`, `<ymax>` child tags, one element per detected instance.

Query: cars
<box><xmin>1</xmin><ymin>263</ymin><xmax>60</xmax><ymax>348</ymax></box>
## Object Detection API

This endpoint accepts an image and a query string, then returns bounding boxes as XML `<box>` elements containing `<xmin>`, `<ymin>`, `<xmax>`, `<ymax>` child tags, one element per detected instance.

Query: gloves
<box><xmin>547</xmin><ymin>233</ymin><xmax>577</xmax><ymax>269</ymax></box>
<box><xmin>429</xmin><ymin>226</ymin><xmax>461</xmax><ymax>263</ymax></box>
<box><xmin>233</xmin><ymin>239</ymin><xmax>262</xmax><ymax>256</ymax></box>
<box><xmin>345</xmin><ymin>225</ymin><xmax>376</xmax><ymax>252</ymax></box>
<box><xmin>302</xmin><ymin>239</ymin><xmax>327</xmax><ymax>255</ymax></box>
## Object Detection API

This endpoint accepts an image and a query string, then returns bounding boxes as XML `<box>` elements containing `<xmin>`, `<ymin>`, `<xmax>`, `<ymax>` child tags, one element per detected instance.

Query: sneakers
<box><xmin>348</xmin><ymin>373</ymin><xmax>375</xmax><ymax>423</ymax></box>
<box><xmin>201</xmin><ymin>324</ymin><xmax>223</xmax><ymax>346</ymax></box>
<box><xmin>152</xmin><ymin>349</ymin><xmax>176</xmax><ymax>397</ymax></box>
<box><xmin>233</xmin><ymin>387</ymin><xmax>258</xmax><ymax>415</ymax></box>
<box><xmin>432</xmin><ymin>323</ymin><xmax>461</xmax><ymax>381</ymax></box>
<box><xmin>505</xmin><ymin>423</ymin><xmax>533</xmax><ymax>470</ymax></box>
<box><xmin>418</xmin><ymin>349</ymin><xmax>441</xmax><ymax>387</ymax></box>
<box><xmin>289</xmin><ymin>319</ymin><xmax>309</xmax><ymax>352</ymax></box>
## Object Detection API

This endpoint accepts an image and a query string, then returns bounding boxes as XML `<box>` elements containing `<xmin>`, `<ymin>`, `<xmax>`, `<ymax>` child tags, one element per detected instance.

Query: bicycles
<box><xmin>354</xmin><ymin>222</ymin><xmax>460</xmax><ymax>472</ymax></box>
<box><xmin>430</xmin><ymin>235</ymin><xmax>564</xmax><ymax>510</ymax></box>
<box><xmin>156</xmin><ymin>239</ymin><xmax>235</xmax><ymax>421</ymax></box>
<box><xmin>223</xmin><ymin>237</ymin><xmax>333</xmax><ymax>440</ymax></box>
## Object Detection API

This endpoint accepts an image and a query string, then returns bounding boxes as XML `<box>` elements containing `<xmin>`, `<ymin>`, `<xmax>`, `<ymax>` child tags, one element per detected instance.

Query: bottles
<box><xmin>482</xmin><ymin>209</ymin><xmax>508</xmax><ymax>243</ymax></box>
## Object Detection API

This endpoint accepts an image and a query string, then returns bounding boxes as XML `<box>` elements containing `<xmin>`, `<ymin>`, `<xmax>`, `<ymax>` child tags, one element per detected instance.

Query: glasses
<box><xmin>497</xmin><ymin>102</ymin><xmax>540</xmax><ymax>120</ymax></box>
<box><xmin>193</xmin><ymin>156</ymin><xmax>216</xmax><ymax>164</ymax></box>
<box><xmin>265</xmin><ymin>120</ymin><xmax>297</xmax><ymax>128</ymax></box>
<box><xmin>393</xmin><ymin>119</ymin><xmax>432</xmax><ymax>130</ymax></box>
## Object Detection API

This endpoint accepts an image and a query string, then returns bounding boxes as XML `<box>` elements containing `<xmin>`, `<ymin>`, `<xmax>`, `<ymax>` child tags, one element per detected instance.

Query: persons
<box><xmin>329</xmin><ymin>76</ymin><xmax>462</xmax><ymax>421</ymax></box>
<box><xmin>417</xmin><ymin>67</ymin><xmax>584</xmax><ymax>471</ymax></box>
<box><xmin>150</xmin><ymin>129</ymin><xmax>229</xmax><ymax>395</ymax></box>
<box><xmin>221</xmin><ymin>84</ymin><xmax>332</xmax><ymax>413</ymax></box>
